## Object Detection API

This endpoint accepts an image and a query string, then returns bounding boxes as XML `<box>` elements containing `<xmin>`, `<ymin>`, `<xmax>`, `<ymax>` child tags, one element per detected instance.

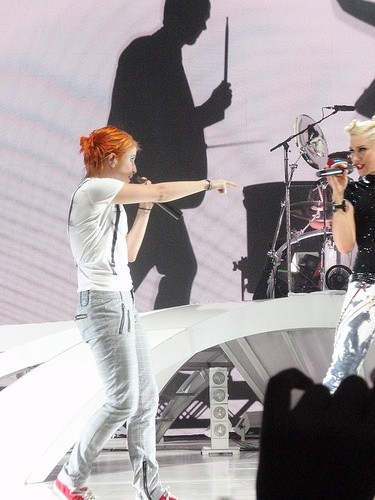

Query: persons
<box><xmin>256</xmin><ymin>368</ymin><xmax>375</xmax><ymax>500</ymax></box>
<box><xmin>322</xmin><ymin>114</ymin><xmax>375</xmax><ymax>393</ymax></box>
<box><xmin>53</xmin><ymin>125</ymin><xmax>238</xmax><ymax>500</ymax></box>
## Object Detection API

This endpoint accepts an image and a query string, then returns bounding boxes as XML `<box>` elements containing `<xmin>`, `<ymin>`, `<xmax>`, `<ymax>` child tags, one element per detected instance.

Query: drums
<box><xmin>322</xmin><ymin>234</ymin><xmax>359</xmax><ymax>294</ymax></box>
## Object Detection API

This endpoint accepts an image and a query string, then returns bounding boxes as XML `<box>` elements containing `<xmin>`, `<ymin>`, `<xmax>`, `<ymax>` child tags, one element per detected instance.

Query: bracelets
<box><xmin>331</xmin><ymin>199</ymin><xmax>347</xmax><ymax>213</ymax></box>
<box><xmin>138</xmin><ymin>206</ymin><xmax>152</xmax><ymax>211</ymax></box>
<box><xmin>207</xmin><ymin>179</ymin><xmax>212</xmax><ymax>191</ymax></box>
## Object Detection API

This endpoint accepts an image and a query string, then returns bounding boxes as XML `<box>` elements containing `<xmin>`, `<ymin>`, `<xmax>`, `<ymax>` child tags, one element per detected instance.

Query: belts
<box><xmin>350</xmin><ymin>272</ymin><xmax>375</xmax><ymax>283</ymax></box>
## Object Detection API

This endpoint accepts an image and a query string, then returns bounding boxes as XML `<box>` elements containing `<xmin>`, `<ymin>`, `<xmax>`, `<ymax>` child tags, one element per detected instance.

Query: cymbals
<box><xmin>293</xmin><ymin>113</ymin><xmax>329</xmax><ymax>169</ymax></box>
<box><xmin>289</xmin><ymin>200</ymin><xmax>334</xmax><ymax>222</ymax></box>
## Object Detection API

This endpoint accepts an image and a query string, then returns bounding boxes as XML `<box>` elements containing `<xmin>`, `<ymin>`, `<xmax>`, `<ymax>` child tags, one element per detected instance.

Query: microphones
<box><xmin>135</xmin><ymin>178</ymin><xmax>183</xmax><ymax>220</ymax></box>
<box><xmin>328</xmin><ymin>105</ymin><xmax>355</xmax><ymax>111</ymax></box>
<box><xmin>315</xmin><ymin>164</ymin><xmax>353</xmax><ymax>177</ymax></box>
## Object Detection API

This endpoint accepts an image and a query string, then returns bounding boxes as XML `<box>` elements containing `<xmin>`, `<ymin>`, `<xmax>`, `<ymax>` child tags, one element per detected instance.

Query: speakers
<box><xmin>208</xmin><ymin>366</ymin><xmax>229</xmax><ymax>450</ymax></box>
<box><xmin>252</xmin><ymin>233</ymin><xmax>327</xmax><ymax>300</ymax></box>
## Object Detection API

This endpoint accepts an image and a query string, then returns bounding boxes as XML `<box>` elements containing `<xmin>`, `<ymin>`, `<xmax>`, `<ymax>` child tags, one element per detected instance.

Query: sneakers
<box><xmin>159</xmin><ymin>489</ymin><xmax>177</xmax><ymax>500</ymax></box>
<box><xmin>53</xmin><ymin>479</ymin><xmax>96</xmax><ymax>500</ymax></box>
